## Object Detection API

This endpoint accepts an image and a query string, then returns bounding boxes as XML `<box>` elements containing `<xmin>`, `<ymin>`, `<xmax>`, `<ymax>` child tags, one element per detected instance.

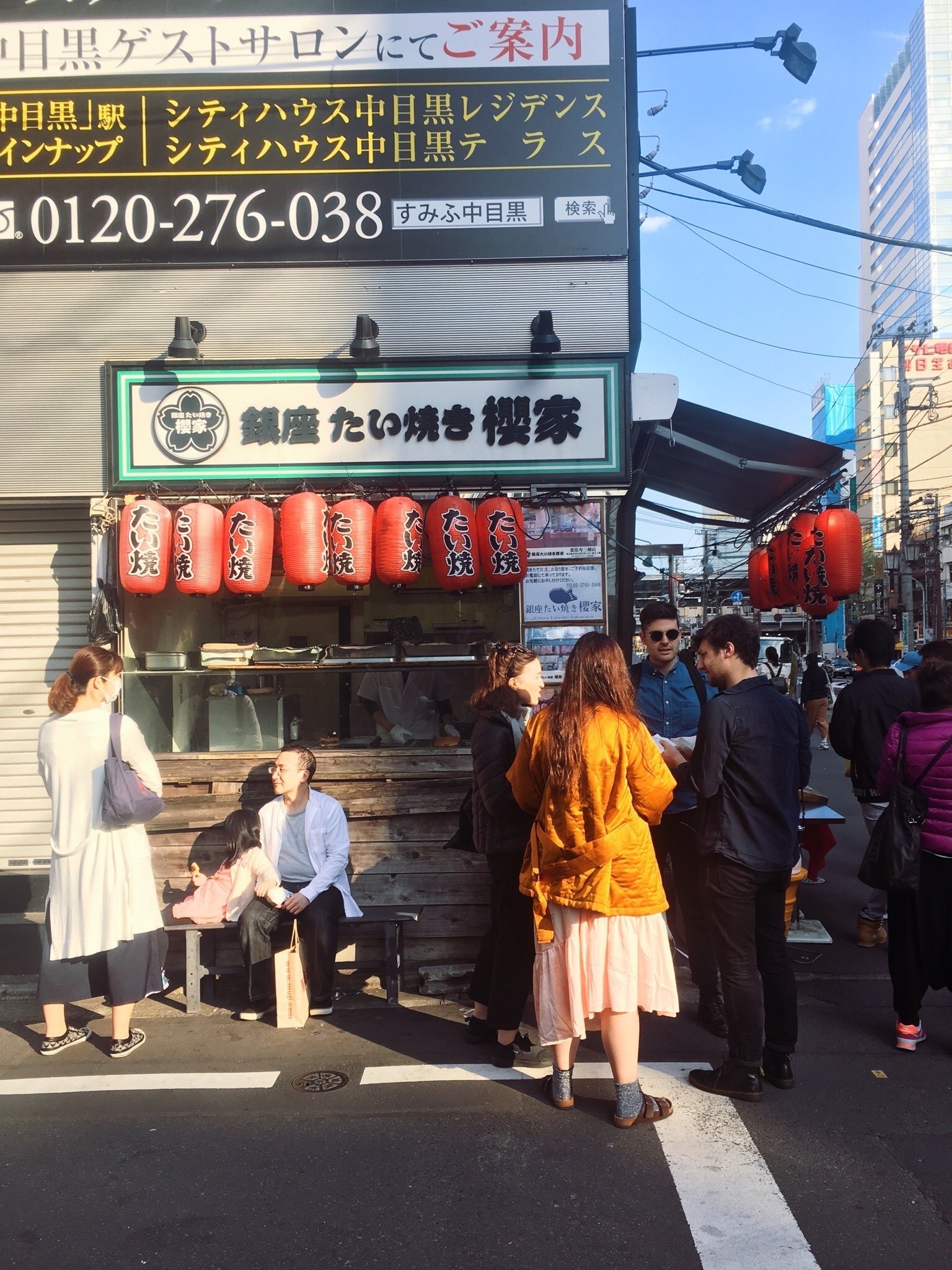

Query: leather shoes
<box><xmin>688</xmin><ymin>1056</ymin><xmax>762</xmax><ymax>1102</ymax></box>
<box><xmin>761</xmin><ymin>1045</ymin><xmax>795</xmax><ymax>1089</ymax></box>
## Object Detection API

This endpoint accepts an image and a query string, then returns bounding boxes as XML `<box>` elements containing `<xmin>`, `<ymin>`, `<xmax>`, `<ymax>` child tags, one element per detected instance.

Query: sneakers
<box><xmin>309</xmin><ymin>996</ymin><xmax>333</xmax><ymax>1015</ymax></box>
<box><xmin>41</xmin><ymin>1025</ymin><xmax>92</xmax><ymax>1055</ymax></box>
<box><xmin>895</xmin><ymin>1015</ymin><xmax>926</xmax><ymax>1050</ymax></box>
<box><xmin>110</xmin><ymin>1028</ymin><xmax>146</xmax><ymax>1058</ymax></box>
<box><xmin>240</xmin><ymin>998</ymin><xmax>277</xmax><ymax>1020</ymax></box>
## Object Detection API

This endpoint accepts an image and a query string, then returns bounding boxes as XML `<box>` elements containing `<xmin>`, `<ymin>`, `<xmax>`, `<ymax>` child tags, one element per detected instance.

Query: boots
<box><xmin>697</xmin><ymin>986</ymin><xmax>729</xmax><ymax>1037</ymax></box>
<box><xmin>856</xmin><ymin>917</ymin><xmax>887</xmax><ymax>947</ymax></box>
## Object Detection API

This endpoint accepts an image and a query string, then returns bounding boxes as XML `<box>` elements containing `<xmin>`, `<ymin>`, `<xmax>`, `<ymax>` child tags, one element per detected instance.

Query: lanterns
<box><xmin>748</xmin><ymin>506</ymin><xmax>863</xmax><ymax>620</ymax></box>
<box><xmin>118</xmin><ymin>491</ymin><xmax>528</xmax><ymax>600</ymax></box>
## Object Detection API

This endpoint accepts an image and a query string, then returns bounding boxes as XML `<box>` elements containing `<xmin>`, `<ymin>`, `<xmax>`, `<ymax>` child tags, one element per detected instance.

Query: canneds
<box><xmin>535</xmin><ymin>645</ymin><xmax>575</xmax><ymax>654</ymax></box>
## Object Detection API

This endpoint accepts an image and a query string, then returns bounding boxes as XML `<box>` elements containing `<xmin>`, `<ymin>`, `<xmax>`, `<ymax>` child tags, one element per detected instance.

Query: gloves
<box><xmin>389</xmin><ymin>723</ymin><xmax>413</xmax><ymax>744</ymax></box>
<box><xmin>443</xmin><ymin>726</ymin><xmax>467</xmax><ymax>740</ymax></box>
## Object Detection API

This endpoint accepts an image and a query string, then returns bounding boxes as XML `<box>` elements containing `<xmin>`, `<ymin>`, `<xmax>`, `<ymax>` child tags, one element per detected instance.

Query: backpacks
<box><xmin>857</xmin><ymin>736</ymin><xmax>952</xmax><ymax>890</ymax></box>
<box><xmin>764</xmin><ymin>662</ymin><xmax>788</xmax><ymax>695</ymax></box>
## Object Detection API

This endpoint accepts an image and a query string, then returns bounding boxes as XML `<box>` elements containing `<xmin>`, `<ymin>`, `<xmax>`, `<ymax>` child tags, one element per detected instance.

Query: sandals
<box><xmin>615</xmin><ymin>1093</ymin><xmax>673</xmax><ymax>1129</ymax></box>
<box><xmin>540</xmin><ymin>1074</ymin><xmax>575</xmax><ymax>1109</ymax></box>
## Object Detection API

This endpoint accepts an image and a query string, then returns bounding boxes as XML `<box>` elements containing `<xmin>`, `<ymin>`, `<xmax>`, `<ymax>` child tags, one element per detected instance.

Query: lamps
<box><xmin>530</xmin><ymin>310</ymin><xmax>561</xmax><ymax>353</ymax></box>
<box><xmin>350</xmin><ymin>314</ymin><xmax>380</xmax><ymax>358</ymax></box>
<box><xmin>639</xmin><ymin>148</ymin><xmax>769</xmax><ymax>196</ymax></box>
<box><xmin>634</xmin><ymin>21</ymin><xmax>818</xmax><ymax>84</ymax></box>
<box><xmin>167</xmin><ymin>314</ymin><xmax>207</xmax><ymax>359</ymax></box>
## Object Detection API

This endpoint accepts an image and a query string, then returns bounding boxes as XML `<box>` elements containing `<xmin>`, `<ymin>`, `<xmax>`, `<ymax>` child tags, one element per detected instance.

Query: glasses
<box><xmin>504</xmin><ymin>642</ymin><xmax>527</xmax><ymax>679</ymax></box>
<box><xmin>268</xmin><ymin>768</ymin><xmax>307</xmax><ymax>776</ymax></box>
<box><xmin>645</xmin><ymin>630</ymin><xmax>680</xmax><ymax>641</ymax></box>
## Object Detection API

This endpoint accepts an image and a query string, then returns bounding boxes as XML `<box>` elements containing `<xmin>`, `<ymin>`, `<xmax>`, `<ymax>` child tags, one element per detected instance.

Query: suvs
<box><xmin>802</xmin><ymin>654</ymin><xmax>824</xmax><ymax>673</ymax></box>
<box><xmin>755</xmin><ymin>637</ymin><xmax>794</xmax><ymax>688</ymax></box>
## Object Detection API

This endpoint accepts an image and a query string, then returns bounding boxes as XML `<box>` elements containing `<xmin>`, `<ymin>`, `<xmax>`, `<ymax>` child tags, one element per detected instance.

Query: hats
<box><xmin>895</xmin><ymin>650</ymin><xmax>923</xmax><ymax>671</ymax></box>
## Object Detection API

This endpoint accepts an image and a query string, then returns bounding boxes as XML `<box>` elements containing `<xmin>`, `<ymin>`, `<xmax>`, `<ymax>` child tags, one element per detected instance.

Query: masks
<box><xmin>97</xmin><ymin>675</ymin><xmax>122</xmax><ymax>703</ymax></box>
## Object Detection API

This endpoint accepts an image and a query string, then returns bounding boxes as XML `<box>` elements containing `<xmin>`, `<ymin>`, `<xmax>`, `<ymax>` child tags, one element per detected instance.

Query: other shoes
<box><xmin>490</xmin><ymin>1037</ymin><xmax>516</xmax><ymax>1068</ymax></box>
<box><xmin>816</xmin><ymin>741</ymin><xmax>829</xmax><ymax>750</ymax></box>
<box><xmin>464</xmin><ymin>1015</ymin><xmax>498</xmax><ymax>1044</ymax></box>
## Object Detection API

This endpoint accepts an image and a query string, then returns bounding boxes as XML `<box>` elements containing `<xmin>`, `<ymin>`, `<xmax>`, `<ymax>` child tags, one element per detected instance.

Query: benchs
<box><xmin>163</xmin><ymin>912</ymin><xmax>418</xmax><ymax>1014</ymax></box>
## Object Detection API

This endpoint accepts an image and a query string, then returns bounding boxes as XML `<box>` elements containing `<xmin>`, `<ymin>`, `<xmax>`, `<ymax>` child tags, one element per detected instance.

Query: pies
<box><xmin>433</xmin><ymin>736</ymin><xmax>460</xmax><ymax>746</ymax></box>
<box><xmin>318</xmin><ymin>737</ymin><xmax>340</xmax><ymax>746</ymax></box>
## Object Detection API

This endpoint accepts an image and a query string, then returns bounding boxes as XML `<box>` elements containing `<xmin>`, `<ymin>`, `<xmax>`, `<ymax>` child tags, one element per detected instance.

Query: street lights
<box><xmin>883</xmin><ymin>570</ymin><xmax>926</xmax><ymax>646</ymax></box>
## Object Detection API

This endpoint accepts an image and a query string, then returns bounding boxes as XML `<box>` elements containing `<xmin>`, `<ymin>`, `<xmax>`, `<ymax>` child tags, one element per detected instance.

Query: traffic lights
<box><xmin>889</xmin><ymin>571</ymin><xmax>894</xmax><ymax>594</ymax></box>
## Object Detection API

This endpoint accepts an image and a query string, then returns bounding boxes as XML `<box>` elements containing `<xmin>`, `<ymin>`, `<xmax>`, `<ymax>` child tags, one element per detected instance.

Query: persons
<box><xmin>659</xmin><ymin>613</ymin><xmax>813</xmax><ymax>1103</ymax></box>
<box><xmin>891</xmin><ymin>640</ymin><xmax>952</xmax><ymax>683</ymax></box>
<box><xmin>239</xmin><ymin>744</ymin><xmax>364</xmax><ymax>1021</ymax></box>
<box><xmin>37</xmin><ymin>646</ymin><xmax>168</xmax><ymax>1058</ymax></box>
<box><xmin>442</xmin><ymin>640</ymin><xmax>544</xmax><ymax>1068</ymax></box>
<box><xmin>161</xmin><ymin>808</ymin><xmax>282</xmax><ymax>925</ymax></box>
<box><xmin>356</xmin><ymin>612</ymin><xmax>462</xmax><ymax>746</ymax></box>
<box><xmin>800</xmin><ymin>653</ymin><xmax>837</xmax><ymax>750</ymax></box>
<box><xmin>627</xmin><ymin>601</ymin><xmax>729</xmax><ymax>1038</ymax></box>
<box><xmin>857</xmin><ymin>656</ymin><xmax>952</xmax><ymax>1051</ymax></box>
<box><xmin>757</xmin><ymin>647</ymin><xmax>790</xmax><ymax>697</ymax></box>
<box><xmin>828</xmin><ymin>618</ymin><xmax>913</xmax><ymax>948</ymax></box>
<box><xmin>505</xmin><ymin>631</ymin><xmax>677</xmax><ymax>1129</ymax></box>
<box><xmin>687</xmin><ymin>646</ymin><xmax>701</xmax><ymax>666</ymax></box>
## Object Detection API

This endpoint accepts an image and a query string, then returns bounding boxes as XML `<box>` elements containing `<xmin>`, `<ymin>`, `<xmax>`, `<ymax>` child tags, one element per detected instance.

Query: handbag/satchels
<box><xmin>274</xmin><ymin>918</ymin><xmax>311</xmax><ymax>1028</ymax></box>
<box><xmin>101</xmin><ymin>712</ymin><xmax>165</xmax><ymax>824</ymax></box>
<box><xmin>824</xmin><ymin>668</ymin><xmax>835</xmax><ymax>705</ymax></box>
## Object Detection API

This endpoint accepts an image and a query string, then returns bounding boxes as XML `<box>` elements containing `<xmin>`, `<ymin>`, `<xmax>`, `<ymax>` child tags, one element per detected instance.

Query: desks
<box><xmin>785</xmin><ymin>799</ymin><xmax>846</xmax><ymax>944</ymax></box>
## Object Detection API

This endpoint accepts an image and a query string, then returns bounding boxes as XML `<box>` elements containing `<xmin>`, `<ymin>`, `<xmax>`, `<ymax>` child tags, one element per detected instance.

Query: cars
<box><xmin>822</xmin><ymin>658</ymin><xmax>862</xmax><ymax>678</ymax></box>
